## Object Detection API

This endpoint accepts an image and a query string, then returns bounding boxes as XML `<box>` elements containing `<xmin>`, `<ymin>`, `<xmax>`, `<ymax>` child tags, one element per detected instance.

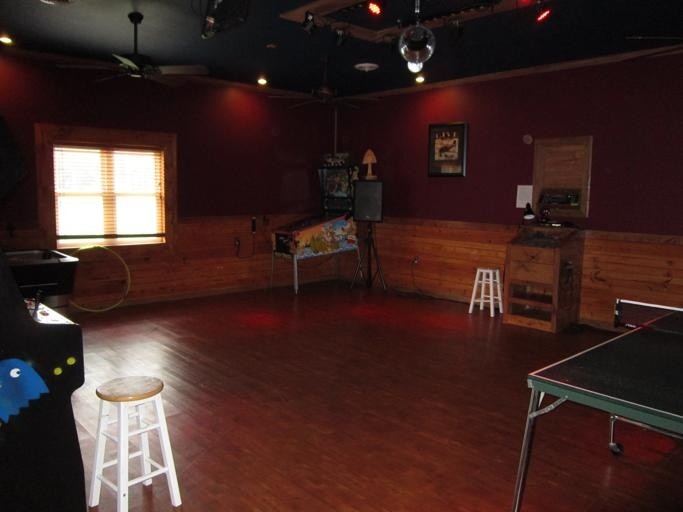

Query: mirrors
<box><xmin>530</xmin><ymin>136</ymin><xmax>592</xmax><ymax>222</ymax></box>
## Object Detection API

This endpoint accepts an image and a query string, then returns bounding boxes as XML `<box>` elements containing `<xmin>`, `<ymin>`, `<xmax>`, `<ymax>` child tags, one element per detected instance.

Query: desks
<box><xmin>514</xmin><ymin>293</ymin><xmax>681</xmax><ymax>510</ymax></box>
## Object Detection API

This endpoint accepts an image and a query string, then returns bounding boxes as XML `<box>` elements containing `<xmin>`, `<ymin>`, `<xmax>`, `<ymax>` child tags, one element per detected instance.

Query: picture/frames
<box><xmin>427</xmin><ymin>121</ymin><xmax>467</xmax><ymax>177</ymax></box>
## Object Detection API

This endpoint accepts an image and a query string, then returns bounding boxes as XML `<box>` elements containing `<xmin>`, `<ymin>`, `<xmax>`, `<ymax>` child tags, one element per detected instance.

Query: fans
<box><xmin>90</xmin><ymin>10</ymin><xmax>210</xmax><ymax>90</ymax></box>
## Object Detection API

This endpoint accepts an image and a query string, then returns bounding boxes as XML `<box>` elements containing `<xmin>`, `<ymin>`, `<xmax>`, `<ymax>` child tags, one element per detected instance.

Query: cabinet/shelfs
<box><xmin>500</xmin><ymin>225</ymin><xmax>584</xmax><ymax>331</ymax></box>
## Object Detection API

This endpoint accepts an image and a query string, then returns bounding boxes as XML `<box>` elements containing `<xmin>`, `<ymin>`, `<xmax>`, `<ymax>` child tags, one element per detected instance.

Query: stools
<box><xmin>468</xmin><ymin>265</ymin><xmax>503</xmax><ymax>318</ymax></box>
<box><xmin>87</xmin><ymin>376</ymin><xmax>183</xmax><ymax>512</ymax></box>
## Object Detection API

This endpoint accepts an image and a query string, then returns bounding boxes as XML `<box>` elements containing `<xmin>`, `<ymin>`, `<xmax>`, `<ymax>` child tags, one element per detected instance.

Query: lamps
<box><xmin>521</xmin><ymin>201</ymin><xmax>537</xmax><ymax>221</ymax></box>
